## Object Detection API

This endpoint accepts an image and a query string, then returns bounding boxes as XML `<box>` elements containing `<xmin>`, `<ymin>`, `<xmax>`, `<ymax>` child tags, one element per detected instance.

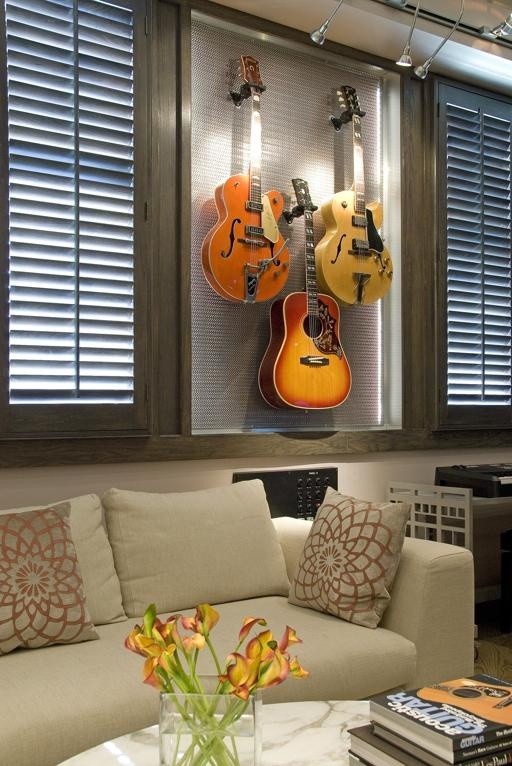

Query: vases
<box><xmin>156</xmin><ymin>695</ymin><xmax>257</xmax><ymax>766</ymax></box>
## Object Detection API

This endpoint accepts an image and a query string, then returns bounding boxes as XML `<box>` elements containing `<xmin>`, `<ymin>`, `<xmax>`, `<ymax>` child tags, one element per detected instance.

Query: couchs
<box><xmin>1</xmin><ymin>480</ymin><xmax>478</xmax><ymax>765</ymax></box>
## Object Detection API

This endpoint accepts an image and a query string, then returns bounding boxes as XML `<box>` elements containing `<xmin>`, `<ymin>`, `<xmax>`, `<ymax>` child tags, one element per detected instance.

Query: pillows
<box><xmin>1</xmin><ymin>493</ymin><xmax>126</xmax><ymax>626</ymax></box>
<box><xmin>0</xmin><ymin>501</ymin><xmax>100</xmax><ymax>654</ymax></box>
<box><xmin>104</xmin><ymin>478</ymin><xmax>291</xmax><ymax>618</ymax></box>
<box><xmin>287</xmin><ymin>486</ymin><xmax>412</xmax><ymax>628</ymax></box>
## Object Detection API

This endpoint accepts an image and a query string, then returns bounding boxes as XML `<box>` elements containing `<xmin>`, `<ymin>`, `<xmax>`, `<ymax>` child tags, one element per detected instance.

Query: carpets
<box><xmin>474</xmin><ymin>640</ymin><xmax>511</xmax><ymax>685</ymax></box>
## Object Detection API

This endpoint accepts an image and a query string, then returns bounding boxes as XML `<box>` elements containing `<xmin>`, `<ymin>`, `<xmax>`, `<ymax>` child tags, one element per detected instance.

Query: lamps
<box><xmin>307</xmin><ymin>1</ymin><xmax>343</xmax><ymax>45</ymax></box>
<box><xmin>412</xmin><ymin>1</ymin><xmax>466</xmax><ymax>79</ymax></box>
<box><xmin>394</xmin><ymin>0</ymin><xmax>422</xmax><ymax>68</ymax></box>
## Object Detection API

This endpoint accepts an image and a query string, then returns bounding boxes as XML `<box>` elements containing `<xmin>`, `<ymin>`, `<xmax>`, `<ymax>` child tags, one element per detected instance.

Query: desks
<box><xmin>387</xmin><ymin>483</ymin><xmax>512</xmax><ymax>604</ymax></box>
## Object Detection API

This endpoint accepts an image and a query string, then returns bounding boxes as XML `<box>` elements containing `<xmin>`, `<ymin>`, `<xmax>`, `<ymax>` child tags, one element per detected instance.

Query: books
<box><xmin>348</xmin><ymin>673</ymin><xmax>512</xmax><ymax>766</ymax></box>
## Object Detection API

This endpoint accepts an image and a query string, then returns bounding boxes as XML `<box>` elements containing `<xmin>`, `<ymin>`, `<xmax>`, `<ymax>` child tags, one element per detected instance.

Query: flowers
<box><xmin>123</xmin><ymin>602</ymin><xmax>307</xmax><ymax>766</ymax></box>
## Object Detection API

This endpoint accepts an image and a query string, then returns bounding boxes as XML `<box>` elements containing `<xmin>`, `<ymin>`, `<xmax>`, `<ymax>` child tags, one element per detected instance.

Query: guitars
<box><xmin>314</xmin><ymin>85</ymin><xmax>392</xmax><ymax>304</ymax></box>
<box><xmin>257</xmin><ymin>178</ymin><xmax>352</xmax><ymax>411</ymax></box>
<box><xmin>200</xmin><ymin>55</ymin><xmax>290</xmax><ymax>305</ymax></box>
